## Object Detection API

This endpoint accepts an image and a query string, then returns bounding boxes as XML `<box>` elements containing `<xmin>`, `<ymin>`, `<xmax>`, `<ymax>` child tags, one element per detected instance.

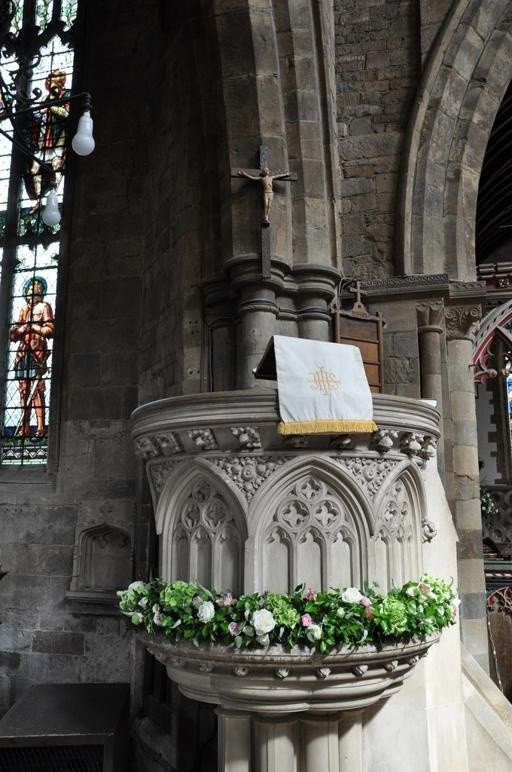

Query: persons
<box><xmin>31</xmin><ymin>66</ymin><xmax>73</xmax><ymax>154</ymax></box>
<box><xmin>5</xmin><ymin>279</ymin><xmax>56</xmax><ymax>441</ymax></box>
<box><xmin>241</xmin><ymin>168</ymin><xmax>291</xmax><ymax>223</ymax></box>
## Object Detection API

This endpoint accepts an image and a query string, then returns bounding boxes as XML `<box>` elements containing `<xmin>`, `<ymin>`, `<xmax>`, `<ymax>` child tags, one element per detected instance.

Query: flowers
<box><xmin>116</xmin><ymin>577</ymin><xmax>462</xmax><ymax>655</ymax></box>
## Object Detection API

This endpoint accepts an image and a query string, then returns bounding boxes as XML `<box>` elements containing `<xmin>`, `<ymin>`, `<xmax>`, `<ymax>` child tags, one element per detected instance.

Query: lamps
<box><xmin>0</xmin><ymin>92</ymin><xmax>96</xmax><ymax>225</ymax></box>
